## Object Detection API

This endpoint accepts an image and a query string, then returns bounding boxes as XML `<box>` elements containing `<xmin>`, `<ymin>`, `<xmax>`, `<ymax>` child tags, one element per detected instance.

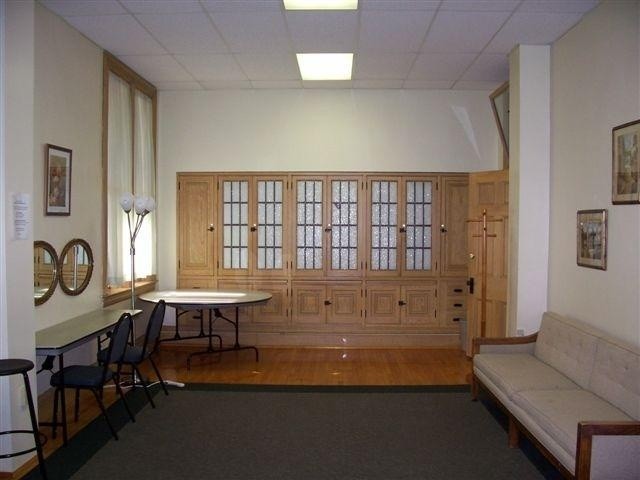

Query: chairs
<box><xmin>97</xmin><ymin>297</ymin><xmax>171</xmax><ymax>407</ymax></box>
<box><xmin>49</xmin><ymin>313</ymin><xmax>137</xmax><ymax>441</ymax></box>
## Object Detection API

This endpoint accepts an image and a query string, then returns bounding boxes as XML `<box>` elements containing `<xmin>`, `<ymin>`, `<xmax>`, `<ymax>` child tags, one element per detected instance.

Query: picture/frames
<box><xmin>611</xmin><ymin>117</ymin><xmax>640</xmax><ymax>204</ymax></box>
<box><xmin>577</xmin><ymin>209</ymin><xmax>608</xmax><ymax>270</ymax></box>
<box><xmin>488</xmin><ymin>83</ymin><xmax>510</xmax><ymax>152</ymax></box>
<box><xmin>46</xmin><ymin>144</ymin><xmax>73</xmax><ymax>216</ymax></box>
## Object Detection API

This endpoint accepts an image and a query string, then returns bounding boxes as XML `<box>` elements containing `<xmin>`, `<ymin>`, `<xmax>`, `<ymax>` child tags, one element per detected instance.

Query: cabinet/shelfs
<box><xmin>289</xmin><ymin>279</ymin><xmax>361</xmax><ymax>333</ymax></box>
<box><xmin>290</xmin><ymin>172</ymin><xmax>366</xmax><ymax>277</ymax></box>
<box><xmin>177</xmin><ymin>172</ymin><xmax>216</xmax><ymax>276</ymax></box>
<box><xmin>438</xmin><ymin>172</ymin><xmax>467</xmax><ymax>277</ymax></box>
<box><xmin>176</xmin><ymin>276</ymin><xmax>216</xmax><ymax>334</ymax></box>
<box><xmin>365</xmin><ymin>172</ymin><xmax>437</xmax><ymax>278</ymax></box>
<box><xmin>217</xmin><ymin>278</ymin><xmax>289</xmax><ymax>333</ymax></box>
<box><xmin>216</xmin><ymin>172</ymin><xmax>290</xmax><ymax>278</ymax></box>
<box><xmin>362</xmin><ymin>278</ymin><xmax>438</xmax><ymax>334</ymax></box>
<box><xmin>439</xmin><ymin>277</ymin><xmax>465</xmax><ymax>333</ymax></box>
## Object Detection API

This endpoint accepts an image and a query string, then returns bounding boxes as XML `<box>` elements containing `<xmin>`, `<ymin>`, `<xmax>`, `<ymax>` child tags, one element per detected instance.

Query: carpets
<box><xmin>73</xmin><ymin>387</ymin><xmax>548</xmax><ymax>480</ymax></box>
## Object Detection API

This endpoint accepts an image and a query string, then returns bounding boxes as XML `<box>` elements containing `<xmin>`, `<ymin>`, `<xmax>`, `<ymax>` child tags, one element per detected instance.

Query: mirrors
<box><xmin>60</xmin><ymin>238</ymin><xmax>95</xmax><ymax>296</ymax></box>
<box><xmin>33</xmin><ymin>240</ymin><xmax>59</xmax><ymax>303</ymax></box>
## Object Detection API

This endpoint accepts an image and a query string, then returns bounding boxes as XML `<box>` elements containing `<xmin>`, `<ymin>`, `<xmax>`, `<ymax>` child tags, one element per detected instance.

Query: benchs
<box><xmin>470</xmin><ymin>312</ymin><xmax>640</xmax><ymax>480</ymax></box>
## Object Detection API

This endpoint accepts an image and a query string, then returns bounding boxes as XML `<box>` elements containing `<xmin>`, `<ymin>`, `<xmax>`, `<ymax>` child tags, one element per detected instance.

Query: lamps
<box><xmin>117</xmin><ymin>195</ymin><xmax>182</xmax><ymax>388</ymax></box>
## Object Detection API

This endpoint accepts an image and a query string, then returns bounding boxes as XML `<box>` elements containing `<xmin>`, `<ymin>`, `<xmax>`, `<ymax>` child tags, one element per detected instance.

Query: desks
<box><xmin>35</xmin><ymin>308</ymin><xmax>144</xmax><ymax>425</ymax></box>
<box><xmin>140</xmin><ymin>288</ymin><xmax>274</xmax><ymax>370</ymax></box>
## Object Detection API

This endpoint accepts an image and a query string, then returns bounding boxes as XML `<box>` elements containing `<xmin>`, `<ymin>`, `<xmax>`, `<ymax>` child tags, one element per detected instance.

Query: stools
<box><xmin>0</xmin><ymin>350</ymin><xmax>46</xmax><ymax>480</ymax></box>
